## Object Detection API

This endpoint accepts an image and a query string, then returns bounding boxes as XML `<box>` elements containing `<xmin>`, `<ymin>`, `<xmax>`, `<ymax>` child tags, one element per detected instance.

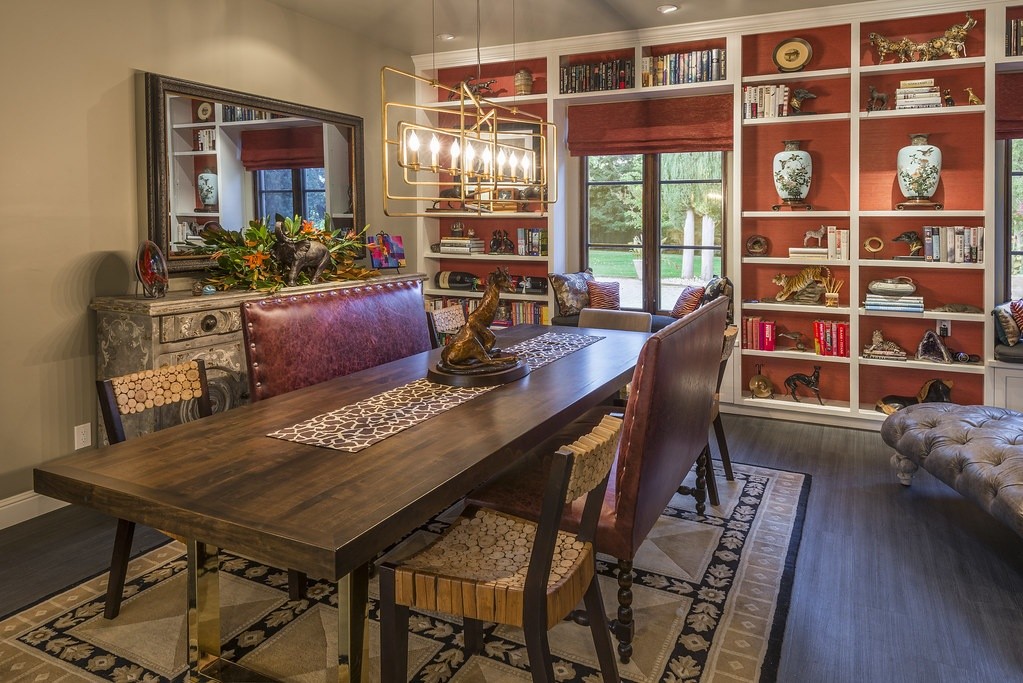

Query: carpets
<box><xmin>0</xmin><ymin>457</ymin><xmax>813</xmax><ymax>683</ymax></box>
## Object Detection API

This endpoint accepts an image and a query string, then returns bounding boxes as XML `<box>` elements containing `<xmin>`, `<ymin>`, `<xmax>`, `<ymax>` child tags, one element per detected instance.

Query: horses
<box><xmin>867</xmin><ymin>32</ymin><xmax>937</xmax><ymax>62</ymax></box>
<box><xmin>867</xmin><ymin>85</ymin><xmax>888</xmax><ymax>107</ymax></box>
<box><xmin>875</xmin><ymin>379</ymin><xmax>953</xmax><ymax>416</ymax></box>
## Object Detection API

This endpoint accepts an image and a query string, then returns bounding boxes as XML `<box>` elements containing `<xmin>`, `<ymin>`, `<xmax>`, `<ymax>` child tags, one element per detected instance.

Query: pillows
<box><xmin>586</xmin><ymin>281</ymin><xmax>621</xmax><ymax>311</ymax></box>
<box><xmin>546</xmin><ymin>268</ymin><xmax>596</xmax><ymax>317</ymax></box>
<box><xmin>990</xmin><ymin>301</ymin><xmax>1022</xmax><ymax>347</ymax></box>
<box><xmin>670</xmin><ymin>285</ymin><xmax>705</xmax><ymax>319</ymax></box>
<box><xmin>702</xmin><ymin>275</ymin><xmax>734</xmax><ymax>329</ymax></box>
<box><xmin>1010</xmin><ymin>298</ymin><xmax>1023</xmax><ymax>334</ymax></box>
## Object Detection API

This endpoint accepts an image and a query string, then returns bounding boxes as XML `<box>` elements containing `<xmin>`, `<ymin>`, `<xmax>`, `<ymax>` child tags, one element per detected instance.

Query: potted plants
<box><xmin>627</xmin><ymin>234</ymin><xmax>680</xmax><ymax>280</ymax></box>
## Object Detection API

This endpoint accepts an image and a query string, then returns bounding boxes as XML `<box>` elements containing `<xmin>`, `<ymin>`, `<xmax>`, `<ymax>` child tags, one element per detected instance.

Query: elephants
<box><xmin>919</xmin><ymin>12</ymin><xmax>978</xmax><ymax>61</ymax></box>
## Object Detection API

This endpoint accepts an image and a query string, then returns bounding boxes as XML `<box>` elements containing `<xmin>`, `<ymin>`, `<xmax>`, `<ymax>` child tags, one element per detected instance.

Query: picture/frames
<box><xmin>454</xmin><ymin>123</ymin><xmax>540</xmax><ymax>199</ymax></box>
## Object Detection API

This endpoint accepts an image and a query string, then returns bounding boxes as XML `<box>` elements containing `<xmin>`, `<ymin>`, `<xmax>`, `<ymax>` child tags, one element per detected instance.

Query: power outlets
<box><xmin>936</xmin><ymin>320</ymin><xmax>951</xmax><ymax>337</ymax></box>
<box><xmin>73</xmin><ymin>422</ymin><xmax>91</xmax><ymax>450</ymax></box>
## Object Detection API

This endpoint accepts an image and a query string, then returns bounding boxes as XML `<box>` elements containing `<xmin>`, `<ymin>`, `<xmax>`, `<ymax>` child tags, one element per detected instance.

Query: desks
<box><xmin>32</xmin><ymin>323</ymin><xmax>656</xmax><ymax>683</ymax></box>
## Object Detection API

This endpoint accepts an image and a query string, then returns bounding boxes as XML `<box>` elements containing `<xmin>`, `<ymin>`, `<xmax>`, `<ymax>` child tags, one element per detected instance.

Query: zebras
<box><xmin>864</xmin><ymin>328</ymin><xmax>905</xmax><ymax>355</ymax></box>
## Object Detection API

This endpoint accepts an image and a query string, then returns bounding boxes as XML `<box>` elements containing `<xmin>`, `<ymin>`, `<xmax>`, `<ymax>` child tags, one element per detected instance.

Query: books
<box><xmin>440</xmin><ymin>237</ymin><xmax>485</xmax><ymax>254</ymax></box>
<box><xmin>193</xmin><ymin>129</ymin><xmax>215</xmax><ymax>150</ymax></box>
<box><xmin>223</xmin><ymin>105</ymin><xmax>289</xmax><ymax>121</ymax></box>
<box><xmin>560</xmin><ymin>59</ymin><xmax>635</xmax><ymax>94</ymax></box>
<box><xmin>1005</xmin><ymin>19</ymin><xmax>1023</xmax><ymax>56</ymax></box>
<box><xmin>424</xmin><ymin>297</ymin><xmax>548</xmax><ymax>345</ymax></box>
<box><xmin>863</xmin><ymin>293</ymin><xmax>925</xmax><ymax>313</ymax></box>
<box><xmin>894</xmin><ymin>79</ymin><xmax>943</xmax><ymax>109</ymax></box>
<box><xmin>923</xmin><ymin>225</ymin><xmax>985</xmax><ymax>264</ymax></box>
<box><xmin>813</xmin><ymin>320</ymin><xmax>850</xmax><ymax>357</ymax></box>
<box><xmin>742</xmin><ymin>85</ymin><xmax>791</xmax><ymax>119</ymax></box>
<box><xmin>517</xmin><ymin>227</ymin><xmax>548</xmax><ymax>256</ymax></box>
<box><xmin>741</xmin><ymin>315</ymin><xmax>777</xmax><ymax>351</ymax></box>
<box><xmin>789</xmin><ymin>226</ymin><xmax>849</xmax><ymax>260</ymax></box>
<box><xmin>177</xmin><ymin>222</ymin><xmax>201</xmax><ymax>243</ymax></box>
<box><xmin>642</xmin><ymin>48</ymin><xmax>726</xmax><ymax>87</ymax></box>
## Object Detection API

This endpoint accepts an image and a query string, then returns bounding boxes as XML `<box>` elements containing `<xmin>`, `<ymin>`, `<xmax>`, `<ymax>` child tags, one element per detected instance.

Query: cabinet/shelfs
<box><xmin>167</xmin><ymin>95</ymin><xmax>353</xmax><ymax>252</ymax></box>
<box><xmin>409</xmin><ymin>0</ymin><xmax>1023</xmax><ymax>432</ymax></box>
<box><xmin>87</xmin><ymin>272</ymin><xmax>430</xmax><ymax>447</ymax></box>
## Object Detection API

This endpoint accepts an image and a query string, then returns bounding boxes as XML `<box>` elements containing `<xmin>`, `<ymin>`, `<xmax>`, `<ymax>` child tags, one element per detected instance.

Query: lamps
<box><xmin>380</xmin><ymin>0</ymin><xmax>559</xmax><ymax>218</ymax></box>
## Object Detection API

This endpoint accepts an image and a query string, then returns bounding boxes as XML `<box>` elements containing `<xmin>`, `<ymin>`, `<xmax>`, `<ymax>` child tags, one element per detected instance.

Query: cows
<box><xmin>272</xmin><ymin>221</ymin><xmax>337</xmax><ymax>286</ymax></box>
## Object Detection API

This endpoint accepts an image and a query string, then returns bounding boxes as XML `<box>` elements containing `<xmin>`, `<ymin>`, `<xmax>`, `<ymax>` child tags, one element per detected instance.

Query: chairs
<box><xmin>373</xmin><ymin>415</ymin><xmax>624</xmax><ymax>683</ymax></box>
<box><xmin>609</xmin><ymin>324</ymin><xmax>738</xmax><ymax>507</ymax></box>
<box><xmin>426</xmin><ymin>304</ymin><xmax>468</xmax><ymax>350</ymax></box>
<box><xmin>93</xmin><ymin>359</ymin><xmax>307</xmax><ymax>620</ymax></box>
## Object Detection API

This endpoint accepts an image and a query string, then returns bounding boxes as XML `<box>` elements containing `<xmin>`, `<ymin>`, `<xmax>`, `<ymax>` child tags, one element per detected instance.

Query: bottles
<box><xmin>514</xmin><ymin>69</ymin><xmax>532</xmax><ymax>95</ymax></box>
<box><xmin>198</xmin><ymin>167</ymin><xmax>218</xmax><ymax>204</ymax></box>
<box><xmin>897</xmin><ymin>132</ymin><xmax>942</xmax><ymax>198</ymax></box>
<box><xmin>434</xmin><ymin>271</ymin><xmax>487</xmax><ymax>289</ymax></box>
<box><xmin>773</xmin><ymin>139</ymin><xmax>812</xmax><ymax>200</ymax></box>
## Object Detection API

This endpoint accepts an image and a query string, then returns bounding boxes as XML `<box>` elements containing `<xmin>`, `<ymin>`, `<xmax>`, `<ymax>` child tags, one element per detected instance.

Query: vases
<box><xmin>771</xmin><ymin>140</ymin><xmax>813</xmax><ymax>211</ymax></box>
<box><xmin>194</xmin><ymin>168</ymin><xmax>218</xmax><ymax>212</ymax></box>
<box><xmin>895</xmin><ymin>134</ymin><xmax>943</xmax><ymax>210</ymax></box>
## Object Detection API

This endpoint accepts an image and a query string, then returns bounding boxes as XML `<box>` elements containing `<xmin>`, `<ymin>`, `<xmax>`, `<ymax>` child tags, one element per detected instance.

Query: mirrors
<box><xmin>145</xmin><ymin>72</ymin><xmax>366</xmax><ymax>279</ymax></box>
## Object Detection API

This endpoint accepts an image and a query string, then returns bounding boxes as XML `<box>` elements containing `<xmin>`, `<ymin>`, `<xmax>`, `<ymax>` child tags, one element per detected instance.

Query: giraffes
<box><xmin>441</xmin><ymin>266</ymin><xmax>518</xmax><ymax>370</ymax></box>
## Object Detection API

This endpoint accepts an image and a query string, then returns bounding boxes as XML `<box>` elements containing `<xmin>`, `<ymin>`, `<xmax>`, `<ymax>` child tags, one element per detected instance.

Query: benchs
<box><xmin>240</xmin><ymin>281</ymin><xmax>433</xmax><ymax>401</ymax></box>
<box><xmin>460</xmin><ymin>295</ymin><xmax>730</xmax><ymax>666</ymax></box>
<box><xmin>881</xmin><ymin>402</ymin><xmax>1023</xmax><ymax>537</ymax></box>
